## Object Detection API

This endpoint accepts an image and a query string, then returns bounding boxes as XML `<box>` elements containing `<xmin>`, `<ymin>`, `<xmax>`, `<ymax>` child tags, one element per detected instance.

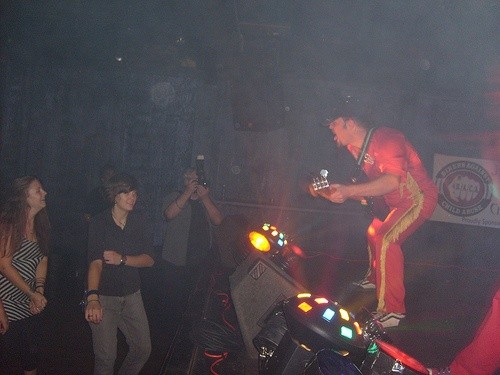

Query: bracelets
<box><xmin>176</xmin><ymin>199</ymin><xmax>184</xmax><ymax>210</ymax></box>
<box><xmin>85</xmin><ymin>290</ymin><xmax>99</xmax><ymax>297</ymax></box>
<box><xmin>36</xmin><ymin>278</ymin><xmax>47</xmax><ymax>287</ymax></box>
<box><xmin>88</xmin><ymin>299</ymin><xmax>100</xmax><ymax>304</ymax></box>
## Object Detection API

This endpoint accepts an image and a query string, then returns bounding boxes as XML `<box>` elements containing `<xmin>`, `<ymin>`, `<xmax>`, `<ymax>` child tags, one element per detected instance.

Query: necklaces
<box><xmin>113</xmin><ymin>216</ymin><xmax>123</xmax><ymax>225</ymax></box>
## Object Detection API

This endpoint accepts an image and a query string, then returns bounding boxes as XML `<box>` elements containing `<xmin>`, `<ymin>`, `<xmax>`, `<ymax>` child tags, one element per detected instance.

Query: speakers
<box><xmin>228</xmin><ymin>249</ymin><xmax>315</xmax><ymax>362</ymax></box>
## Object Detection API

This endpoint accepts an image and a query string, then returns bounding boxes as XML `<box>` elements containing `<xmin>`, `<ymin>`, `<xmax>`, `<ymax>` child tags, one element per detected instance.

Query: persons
<box><xmin>83</xmin><ymin>173</ymin><xmax>157</xmax><ymax>375</ymax></box>
<box><xmin>308</xmin><ymin>103</ymin><xmax>439</xmax><ymax>328</ymax></box>
<box><xmin>0</xmin><ymin>176</ymin><xmax>51</xmax><ymax>375</ymax></box>
<box><xmin>156</xmin><ymin>166</ymin><xmax>225</xmax><ymax>316</ymax></box>
<box><xmin>81</xmin><ymin>165</ymin><xmax>115</xmax><ymax>224</ymax></box>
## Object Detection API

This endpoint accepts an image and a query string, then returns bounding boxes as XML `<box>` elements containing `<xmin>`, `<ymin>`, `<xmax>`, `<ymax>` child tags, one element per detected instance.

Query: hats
<box><xmin>325</xmin><ymin>101</ymin><xmax>365</xmax><ymax>123</ymax></box>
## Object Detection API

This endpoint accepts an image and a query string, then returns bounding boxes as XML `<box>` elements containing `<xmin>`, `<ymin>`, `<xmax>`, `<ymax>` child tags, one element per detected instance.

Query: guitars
<box><xmin>312</xmin><ymin>173</ymin><xmax>390</xmax><ymax>223</ymax></box>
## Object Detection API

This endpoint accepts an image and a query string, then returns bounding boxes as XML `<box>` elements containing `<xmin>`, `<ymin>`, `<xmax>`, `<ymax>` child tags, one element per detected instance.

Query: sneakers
<box><xmin>374</xmin><ymin>312</ymin><xmax>407</xmax><ymax>328</ymax></box>
<box><xmin>352</xmin><ymin>279</ymin><xmax>377</xmax><ymax>289</ymax></box>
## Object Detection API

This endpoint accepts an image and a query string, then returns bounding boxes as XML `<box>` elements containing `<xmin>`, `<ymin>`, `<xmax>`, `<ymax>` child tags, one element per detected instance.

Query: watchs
<box><xmin>121</xmin><ymin>256</ymin><xmax>127</xmax><ymax>265</ymax></box>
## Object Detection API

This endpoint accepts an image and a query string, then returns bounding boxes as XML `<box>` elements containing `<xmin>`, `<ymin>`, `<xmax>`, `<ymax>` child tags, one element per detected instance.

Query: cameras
<box><xmin>192</xmin><ymin>176</ymin><xmax>209</xmax><ymax>192</ymax></box>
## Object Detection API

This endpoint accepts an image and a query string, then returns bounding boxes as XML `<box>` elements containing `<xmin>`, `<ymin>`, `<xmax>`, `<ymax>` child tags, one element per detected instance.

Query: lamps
<box><xmin>248</xmin><ymin>222</ymin><xmax>286</xmax><ymax>253</ymax></box>
<box><xmin>285</xmin><ymin>291</ymin><xmax>371</xmax><ymax>357</ymax></box>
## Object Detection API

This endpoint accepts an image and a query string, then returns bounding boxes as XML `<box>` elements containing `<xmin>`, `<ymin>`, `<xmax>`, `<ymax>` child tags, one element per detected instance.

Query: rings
<box><xmin>88</xmin><ymin>315</ymin><xmax>92</xmax><ymax>317</ymax></box>
